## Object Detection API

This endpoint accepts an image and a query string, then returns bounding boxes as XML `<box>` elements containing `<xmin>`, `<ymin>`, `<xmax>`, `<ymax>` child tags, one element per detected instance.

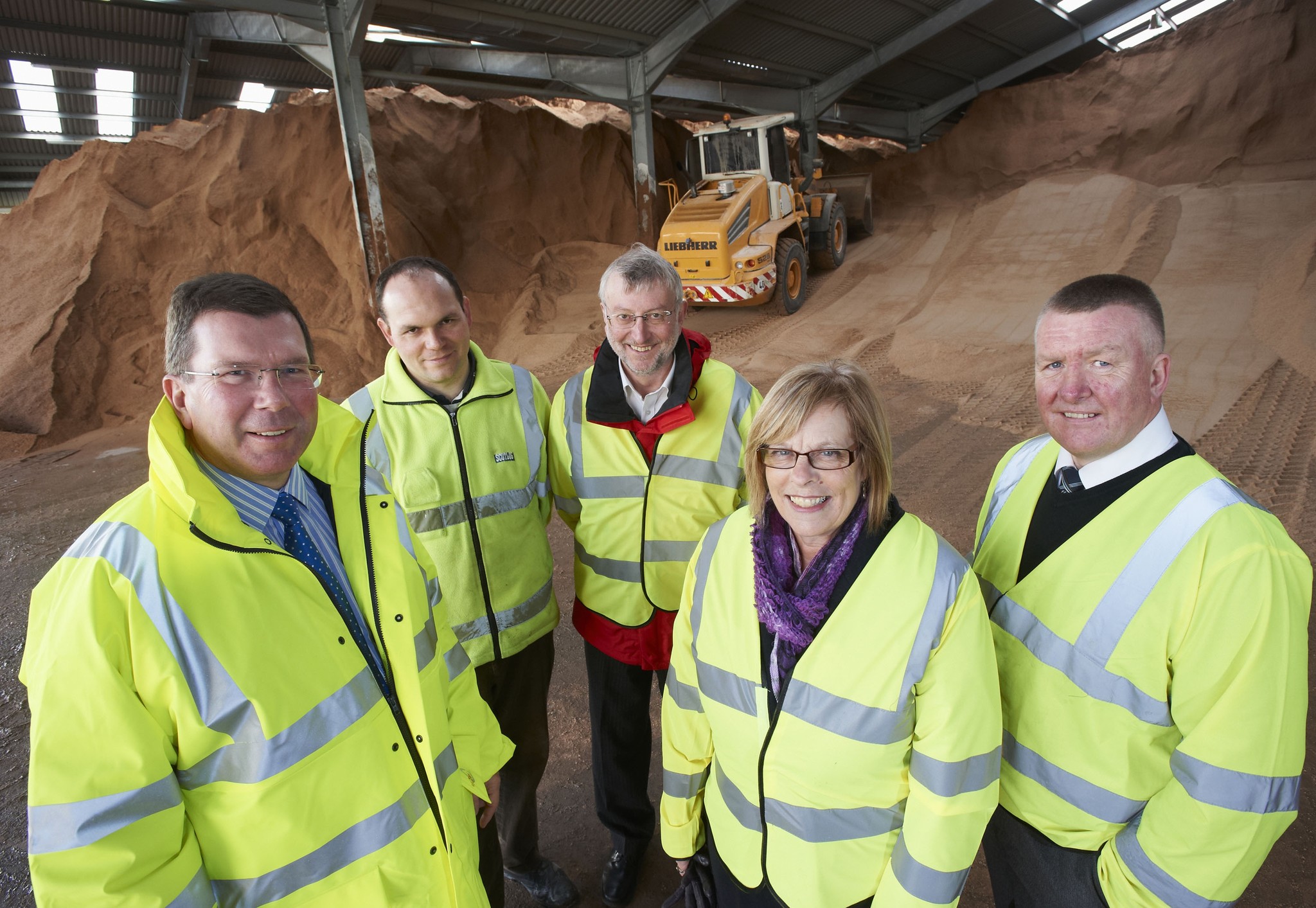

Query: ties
<box><xmin>1056</xmin><ymin>466</ymin><xmax>1084</xmax><ymax>494</ymax></box>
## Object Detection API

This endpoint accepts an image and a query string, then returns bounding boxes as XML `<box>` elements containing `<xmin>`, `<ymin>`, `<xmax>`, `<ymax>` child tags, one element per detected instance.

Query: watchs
<box><xmin>676</xmin><ymin>864</ymin><xmax>686</xmax><ymax>874</ymax></box>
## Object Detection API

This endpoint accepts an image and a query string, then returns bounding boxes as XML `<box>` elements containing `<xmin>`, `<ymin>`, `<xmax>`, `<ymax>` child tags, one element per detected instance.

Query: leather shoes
<box><xmin>598</xmin><ymin>847</ymin><xmax>640</xmax><ymax>908</ymax></box>
<box><xmin>503</xmin><ymin>856</ymin><xmax>579</xmax><ymax>908</ymax></box>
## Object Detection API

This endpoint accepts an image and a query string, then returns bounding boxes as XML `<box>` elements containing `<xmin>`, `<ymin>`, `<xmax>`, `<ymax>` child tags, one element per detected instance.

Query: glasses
<box><xmin>756</xmin><ymin>440</ymin><xmax>863</xmax><ymax>470</ymax></box>
<box><xmin>177</xmin><ymin>363</ymin><xmax>326</xmax><ymax>389</ymax></box>
<box><xmin>603</xmin><ymin>300</ymin><xmax>680</xmax><ymax>329</ymax></box>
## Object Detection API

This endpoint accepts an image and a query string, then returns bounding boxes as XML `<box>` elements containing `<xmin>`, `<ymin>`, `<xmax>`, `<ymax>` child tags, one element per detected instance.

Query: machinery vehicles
<box><xmin>658</xmin><ymin>111</ymin><xmax>872</xmax><ymax>317</ymax></box>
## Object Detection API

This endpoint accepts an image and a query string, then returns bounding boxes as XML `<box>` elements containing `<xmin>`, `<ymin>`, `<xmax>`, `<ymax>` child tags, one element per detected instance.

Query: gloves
<box><xmin>661</xmin><ymin>852</ymin><xmax>718</xmax><ymax>908</ymax></box>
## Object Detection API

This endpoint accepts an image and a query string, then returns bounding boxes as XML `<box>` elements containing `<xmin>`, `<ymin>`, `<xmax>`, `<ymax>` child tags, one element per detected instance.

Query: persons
<box><xmin>650</xmin><ymin>359</ymin><xmax>1005</xmax><ymax>908</ymax></box>
<box><xmin>19</xmin><ymin>271</ymin><xmax>516</xmax><ymax>908</ymax></box>
<box><xmin>546</xmin><ymin>242</ymin><xmax>766</xmax><ymax>908</ymax></box>
<box><xmin>338</xmin><ymin>255</ymin><xmax>584</xmax><ymax>907</ymax></box>
<box><xmin>963</xmin><ymin>275</ymin><xmax>1314</xmax><ymax>908</ymax></box>
